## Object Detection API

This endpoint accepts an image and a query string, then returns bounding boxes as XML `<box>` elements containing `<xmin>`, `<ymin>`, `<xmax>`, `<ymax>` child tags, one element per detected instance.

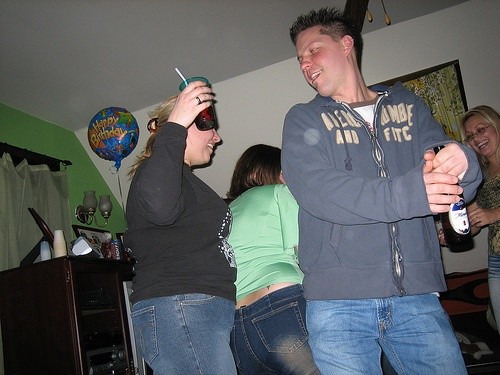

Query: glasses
<box><xmin>467</xmin><ymin>125</ymin><xmax>492</xmax><ymax>142</ymax></box>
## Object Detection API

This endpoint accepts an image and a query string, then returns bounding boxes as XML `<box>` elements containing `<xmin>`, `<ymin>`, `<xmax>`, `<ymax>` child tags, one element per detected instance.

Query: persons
<box><xmin>280</xmin><ymin>7</ymin><xmax>483</xmax><ymax>375</ymax></box>
<box><xmin>227</xmin><ymin>144</ymin><xmax>322</xmax><ymax>375</ymax></box>
<box><xmin>122</xmin><ymin>80</ymin><xmax>238</xmax><ymax>375</ymax></box>
<box><xmin>458</xmin><ymin>104</ymin><xmax>500</xmax><ymax>336</ymax></box>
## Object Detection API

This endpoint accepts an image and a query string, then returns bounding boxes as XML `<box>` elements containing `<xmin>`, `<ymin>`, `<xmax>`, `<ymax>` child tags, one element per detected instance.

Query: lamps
<box><xmin>76</xmin><ymin>190</ymin><xmax>113</xmax><ymax>228</ymax></box>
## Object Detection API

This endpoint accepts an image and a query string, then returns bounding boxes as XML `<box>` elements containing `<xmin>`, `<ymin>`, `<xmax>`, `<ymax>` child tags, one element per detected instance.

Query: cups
<box><xmin>53</xmin><ymin>229</ymin><xmax>66</xmax><ymax>258</ymax></box>
<box><xmin>40</xmin><ymin>241</ymin><xmax>51</xmax><ymax>261</ymax></box>
<box><xmin>100</xmin><ymin>233</ymin><xmax>112</xmax><ymax>260</ymax></box>
<box><xmin>179</xmin><ymin>77</ymin><xmax>216</xmax><ymax>131</ymax></box>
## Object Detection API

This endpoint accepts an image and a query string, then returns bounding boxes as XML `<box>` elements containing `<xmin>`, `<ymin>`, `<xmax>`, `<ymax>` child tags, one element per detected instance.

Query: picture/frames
<box><xmin>71</xmin><ymin>224</ymin><xmax>116</xmax><ymax>259</ymax></box>
<box><xmin>368</xmin><ymin>59</ymin><xmax>474</xmax><ymax>150</ymax></box>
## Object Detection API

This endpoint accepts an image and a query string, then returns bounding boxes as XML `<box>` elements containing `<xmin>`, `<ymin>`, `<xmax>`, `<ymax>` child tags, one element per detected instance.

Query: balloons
<box><xmin>88</xmin><ymin>107</ymin><xmax>139</xmax><ymax>164</ymax></box>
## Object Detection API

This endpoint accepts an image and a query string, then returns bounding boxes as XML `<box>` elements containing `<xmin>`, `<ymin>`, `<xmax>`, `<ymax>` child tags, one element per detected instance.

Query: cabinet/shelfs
<box><xmin>0</xmin><ymin>259</ymin><xmax>140</xmax><ymax>375</ymax></box>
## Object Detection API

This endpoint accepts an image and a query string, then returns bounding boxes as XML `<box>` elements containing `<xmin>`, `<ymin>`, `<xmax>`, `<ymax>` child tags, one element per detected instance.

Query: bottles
<box><xmin>112</xmin><ymin>238</ymin><xmax>124</xmax><ymax>261</ymax></box>
<box><xmin>433</xmin><ymin>145</ymin><xmax>473</xmax><ymax>253</ymax></box>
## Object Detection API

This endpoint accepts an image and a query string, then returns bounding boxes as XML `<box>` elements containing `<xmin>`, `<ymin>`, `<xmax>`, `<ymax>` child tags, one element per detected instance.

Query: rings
<box><xmin>196</xmin><ymin>96</ymin><xmax>201</xmax><ymax>104</ymax></box>
